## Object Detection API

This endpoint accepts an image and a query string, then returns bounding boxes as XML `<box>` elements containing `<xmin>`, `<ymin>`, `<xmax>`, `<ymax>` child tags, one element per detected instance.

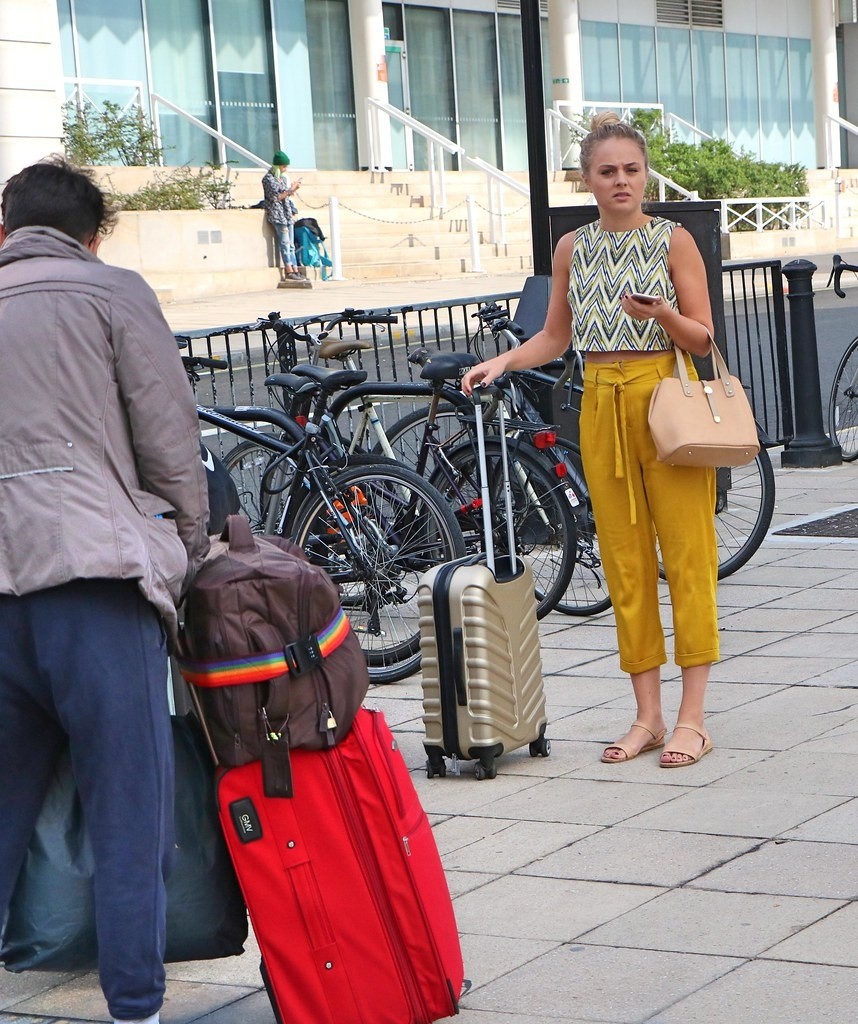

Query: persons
<box><xmin>0</xmin><ymin>164</ymin><xmax>209</xmax><ymax>1023</ymax></box>
<box><xmin>263</xmin><ymin>151</ymin><xmax>307</xmax><ymax>281</ymax></box>
<box><xmin>462</xmin><ymin>113</ymin><xmax>719</xmax><ymax>768</ymax></box>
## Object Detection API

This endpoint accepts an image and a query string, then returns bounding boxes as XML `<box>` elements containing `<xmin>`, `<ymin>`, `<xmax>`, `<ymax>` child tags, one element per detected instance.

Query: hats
<box><xmin>273</xmin><ymin>150</ymin><xmax>290</xmax><ymax>165</ymax></box>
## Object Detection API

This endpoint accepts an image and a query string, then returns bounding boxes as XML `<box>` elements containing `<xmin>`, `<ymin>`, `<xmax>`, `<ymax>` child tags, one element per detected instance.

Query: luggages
<box><xmin>170</xmin><ymin>611</ymin><xmax>466</xmax><ymax>1024</ymax></box>
<box><xmin>416</xmin><ymin>383</ymin><xmax>551</xmax><ymax>781</ymax></box>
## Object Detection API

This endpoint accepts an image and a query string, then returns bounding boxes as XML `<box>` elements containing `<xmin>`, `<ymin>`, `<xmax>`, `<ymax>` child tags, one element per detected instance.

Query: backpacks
<box><xmin>294</xmin><ymin>218</ymin><xmax>332</xmax><ymax>281</ymax></box>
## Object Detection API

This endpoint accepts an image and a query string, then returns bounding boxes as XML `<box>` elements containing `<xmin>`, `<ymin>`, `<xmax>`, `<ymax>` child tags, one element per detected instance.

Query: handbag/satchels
<box><xmin>647</xmin><ymin>325</ymin><xmax>760</xmax><ymax>468</ymax></box>
<box><xmin>181</xmin><ymin>513</ymin><xmax>370</xmax><ymax>799</ymax></box>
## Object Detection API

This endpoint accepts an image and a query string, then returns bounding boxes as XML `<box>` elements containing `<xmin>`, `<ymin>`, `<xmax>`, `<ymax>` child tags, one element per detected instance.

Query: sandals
<box><xmin>659</xmin><ymin>721</ymin><xmax>712</xmax><ymax>768</ymax></box>
<box><xmin>600</xmin><ymin>720</ymin><xmax>667</xmax><ymax>763</ymax></box>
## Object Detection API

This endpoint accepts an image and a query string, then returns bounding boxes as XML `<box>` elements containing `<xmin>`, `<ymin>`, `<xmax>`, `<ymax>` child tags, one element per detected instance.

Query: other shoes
<box><xmin>285</xmin><ymin>271</ymin><xmax>303</xmax><ymax>281</ymax></box>
<box><xmin>294</xmin><ymin>272</ymin><xmax>306</xmax><ymax>280</ymax></box>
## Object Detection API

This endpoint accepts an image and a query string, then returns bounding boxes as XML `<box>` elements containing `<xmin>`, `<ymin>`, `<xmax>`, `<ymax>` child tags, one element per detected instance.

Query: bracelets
<box><xmin>286</xmin><ymin>189</ymin><xmax>293</xmax><ymax>195</ymax></box>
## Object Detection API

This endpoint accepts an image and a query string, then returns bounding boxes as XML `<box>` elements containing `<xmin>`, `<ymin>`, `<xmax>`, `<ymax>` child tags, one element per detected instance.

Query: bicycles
<box><xmin>824</xmin><ymin>253</ymin><xmax>858</xmax><ymax>463</ymax></box>
<box><xmin>173</xmin><ymin>308</ymin><xmax>779</xmax><ymax>687</ymax></box>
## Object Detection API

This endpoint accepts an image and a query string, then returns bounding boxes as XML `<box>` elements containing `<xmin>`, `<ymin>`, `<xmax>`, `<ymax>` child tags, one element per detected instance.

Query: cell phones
<box><xmin>631</xmin><ymin>293</ymin><xmax>659</xmax><ymax>304</ymax></box>
